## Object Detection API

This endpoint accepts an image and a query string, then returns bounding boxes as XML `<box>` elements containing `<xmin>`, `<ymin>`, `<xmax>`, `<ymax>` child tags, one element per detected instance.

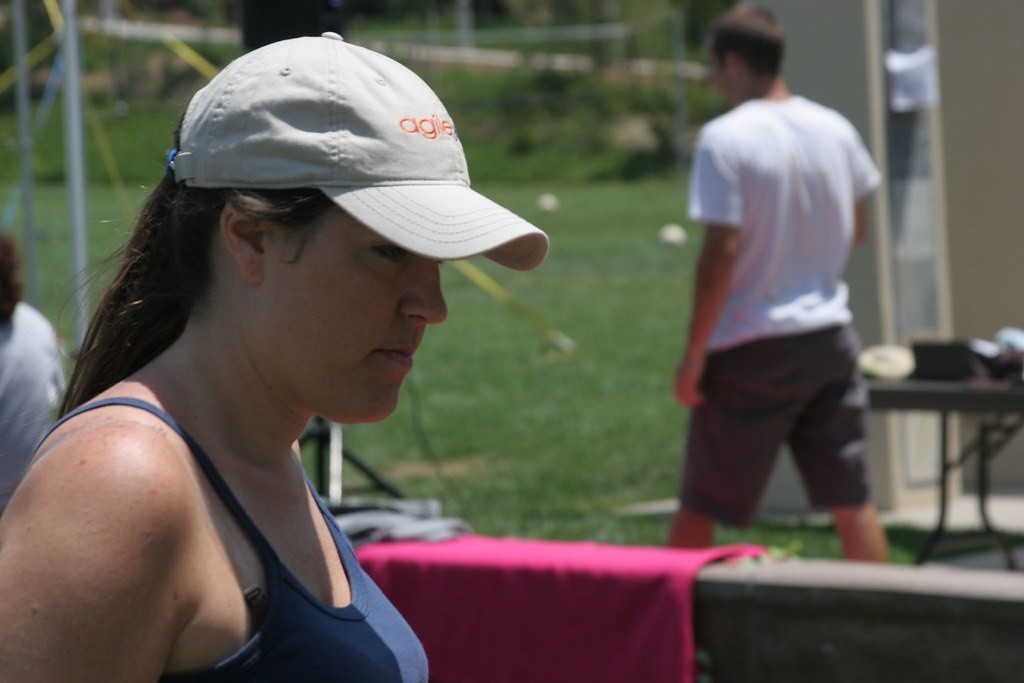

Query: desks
<box><xmin>866</xmin><ymin>376</ymin><xmax>1023</xmax><ymax>574</ymax></box>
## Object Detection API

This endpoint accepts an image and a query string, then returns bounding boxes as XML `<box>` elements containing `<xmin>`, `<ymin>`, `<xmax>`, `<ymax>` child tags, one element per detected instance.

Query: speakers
<box><xmin>241</xmin><ymin>0</ymin><xmax>346</xmax><ymax>52</ymax></box>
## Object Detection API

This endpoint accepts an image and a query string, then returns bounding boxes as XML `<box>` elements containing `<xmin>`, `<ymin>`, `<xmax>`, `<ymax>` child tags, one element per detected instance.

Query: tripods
<box><xmin>296</xmin><ymin>415</ymin><xmax>407</xmax><ymax>509</ymax></box>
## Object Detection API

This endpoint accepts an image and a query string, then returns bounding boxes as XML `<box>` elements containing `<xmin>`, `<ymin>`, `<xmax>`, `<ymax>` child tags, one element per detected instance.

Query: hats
<box><xmin>164</xmin><ymin>32</ymin><xmax>549</xmax><ymax>272</ymax></box>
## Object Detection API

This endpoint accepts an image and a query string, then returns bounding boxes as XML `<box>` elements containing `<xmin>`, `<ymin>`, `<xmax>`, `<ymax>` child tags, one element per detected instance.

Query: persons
<box><xmin>664</xmin><ymin>0</ymin><xmax>890</xmax><ymax>563</ymax></box>
<box><xmin>0</xmin><ymin>233</ymin><xmax>62</xmax><ymax>515</ymax></box>
<box><xmin>0</xmin><ymin>30</ymin><xmax>550</xmax><ymax>683</ymax></box>
